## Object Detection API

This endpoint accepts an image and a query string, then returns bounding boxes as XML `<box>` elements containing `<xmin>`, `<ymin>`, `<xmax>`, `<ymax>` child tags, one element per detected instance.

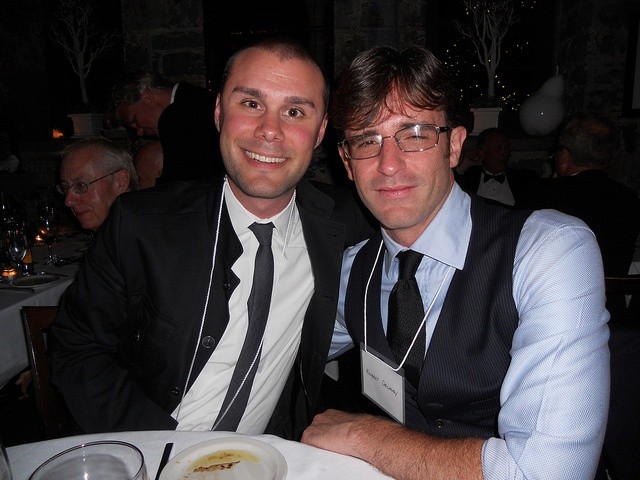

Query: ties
<box><xmin>212</xmin><ymin>222</ymin><xmax>275</xmax><ymax>432</ymax></box>
<box><xmin>385</xmin><ymin>250</ymin><xmax>426</xmax><ymax>392</ymax></box>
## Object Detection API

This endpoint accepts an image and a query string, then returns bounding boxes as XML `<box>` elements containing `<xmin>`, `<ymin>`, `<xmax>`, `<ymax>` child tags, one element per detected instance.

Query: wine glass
<box><xmin>36</xmin><ymin>205</ymin><xmax>65</xmax><ymax>266</ymax></box>
<box><xmin>6</xmin><ymin>232</ymin><xmax>30</xmax><ymax>272</ymax></box>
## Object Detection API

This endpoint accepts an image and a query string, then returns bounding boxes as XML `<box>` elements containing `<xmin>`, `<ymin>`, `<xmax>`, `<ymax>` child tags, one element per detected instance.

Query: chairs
<box><xmin>20</xmin><ymin>306</ymin><xmax>76</xmax><ymax>431</ymax></box>
<box><xmin>604</xmin><ymin>273</ymin><xmax>640</xmax><ymax>480</ymax></box>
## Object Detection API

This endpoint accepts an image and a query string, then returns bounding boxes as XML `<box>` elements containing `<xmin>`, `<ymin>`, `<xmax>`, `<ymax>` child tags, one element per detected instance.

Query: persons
<box><xmin>453</xmin><ymin>127</ymin><xmax>550</xmax><ymax>210</ymax></box>
<box><xmin>0</xmin><ymin>135</ymin><xmax>140</xmax><ymax>448</ymax></box>
<box><xmin>106</xmin><ymin>69</ymin><xmax>227</xmax><ymax>188</ymax></box>
<box><xmin>44</xmin><ymin>41</ymin><xmax>378</xmax><ymax>441</ymax></box>
<box><xmin>134</xmin><ymin>137</ymin><xmax>163</xmax><ymax>187</ymax></box>
<box><xmin>541</xmin><ymin>127</ymin><xmax>640</xmax><ymax>324</ymax></box>
<box><xmin>301</xmin><ymin>42</ymin><xmax>612</xmax><ymax>480</ymax></box>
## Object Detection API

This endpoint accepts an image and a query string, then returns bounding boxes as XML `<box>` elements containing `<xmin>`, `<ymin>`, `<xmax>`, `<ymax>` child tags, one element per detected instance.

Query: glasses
<box><xmin>337</xmin><ymin>124</ymin><xmax>453</xmax><ymax>160</ymax></box>
<box><xmin>57</xmin><ymin>168</ymin><xmax>123</xmax><ymax>193</ymax></box>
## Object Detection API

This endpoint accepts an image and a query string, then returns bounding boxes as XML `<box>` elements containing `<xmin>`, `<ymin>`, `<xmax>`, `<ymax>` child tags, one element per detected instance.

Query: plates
<box><xmin>156</xmin><ymin>436</ymin><xmax>287</xmax><ymax>480</ymax></box>
<box><xmin>9</xmin><ymin>273</ymin><xmax>60</xmax><ymax>288</ymax></box>
